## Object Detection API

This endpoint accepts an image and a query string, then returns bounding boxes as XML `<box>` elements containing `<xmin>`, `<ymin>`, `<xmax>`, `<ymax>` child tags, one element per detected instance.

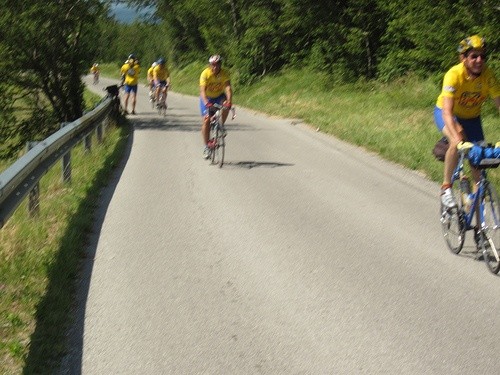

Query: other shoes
<box><xmin>440</xmin><ymin>187</ymin><xmax>457</xmax><ymax>208</ymax></box>
<box><xmin>203</xmin><ymin>146</ymin><xmax>211</xmax><ymax>159</ymax></box>
<box><xmin>475</xmin><ymin>236</ymin><xmax>491</xmax><ymax>249</ymax></box>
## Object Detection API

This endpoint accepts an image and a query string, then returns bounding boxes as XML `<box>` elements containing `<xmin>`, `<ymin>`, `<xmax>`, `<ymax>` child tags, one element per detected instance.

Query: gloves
<box><xmin>456</xmin><ymin>141</ymin><xmax>473</xmax><ymax>155</ymax></box>
<box><xmin>495</xmin><ymin>141</ymin><xmax>500</xmax><ymax>149</ymax></box>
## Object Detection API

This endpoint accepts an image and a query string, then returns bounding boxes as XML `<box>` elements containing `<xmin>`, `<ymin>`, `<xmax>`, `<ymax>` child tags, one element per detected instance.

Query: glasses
<box><xmin>470</xmin><ymin>53</ymin><xmax>487</xmax><ymax>60</ymax></box>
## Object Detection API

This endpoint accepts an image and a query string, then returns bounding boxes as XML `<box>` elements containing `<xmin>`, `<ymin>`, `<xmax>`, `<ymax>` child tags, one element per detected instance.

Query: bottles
<box><xmin>460</xmin><ymin>178</ymin><xmax>471</xmax><ymax>203</ymax></box>
<box><xmin>464</xmin><ymin>193</ymin><xmax>475</xmax><ymax>213</ymax></box>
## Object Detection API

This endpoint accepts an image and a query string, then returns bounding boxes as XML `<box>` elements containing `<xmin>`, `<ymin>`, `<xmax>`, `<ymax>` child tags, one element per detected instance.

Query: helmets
<box><xmin>128</xmin><ymin>54</ymin><xmax>135</xmax><ymax>60</ymax></box>
<box><xmin>151</xmin><ymin>62</ymin><xmax>157</xmax><ymax>68</ymax></box>
<box><xmin>457</xmin><ymin>34</ymin><xmax>486</xmax><ymax>53</ymax></box>
<box><xmin>157</xmin><ymin>58</ymin><xmax>166</xmax><ymax>65</ymax></box>
<box><xmin>209</xmin><ymin>54</ymin><xmax>222</xmax><ymax>64</ymax></box>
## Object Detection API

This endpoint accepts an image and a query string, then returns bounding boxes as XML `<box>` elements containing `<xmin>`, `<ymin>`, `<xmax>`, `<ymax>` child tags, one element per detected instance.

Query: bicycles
<box><xmin>439</xmin><ymin>141</ymin><xmax>500</xmax><ymax>273</ymax></box>
<box><xmin>104</xmin><ymin>84</ymin><xmax>126</xmax><ymax>127</ymax></box>
<box><xmin>148</xmin><ymin>80</ymin><xmax>171</xmax><ymax>115</ymax></box>
<box><xmin>204</xmin><ymin>102</ymin><xmax>236</xmax><ymax>168</ymax></box>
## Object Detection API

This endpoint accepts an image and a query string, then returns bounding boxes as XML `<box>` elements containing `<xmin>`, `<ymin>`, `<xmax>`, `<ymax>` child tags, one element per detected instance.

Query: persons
<box><xmin>198</xmin><ymin>54</ymin><xmax>234</xmax><ymax>160</ymax></box>
<box><xmin>146</xmin><ymin>58</ymin><xmax>170</xmax><ymax>120</ymax></box>
<box><xmin>434</xmin><ymin>33</ymin><xmax>500</xmax><ymax>255</ymax></box>
<box><xmin>89</xmin><ymin>62</ymin><xmax>103</xmax><ymax>82</ymax></box>
<box><xmin>120</xmin><ymin>53</ymin><xmax>141</xmax><ymax>114</ymax></box>
